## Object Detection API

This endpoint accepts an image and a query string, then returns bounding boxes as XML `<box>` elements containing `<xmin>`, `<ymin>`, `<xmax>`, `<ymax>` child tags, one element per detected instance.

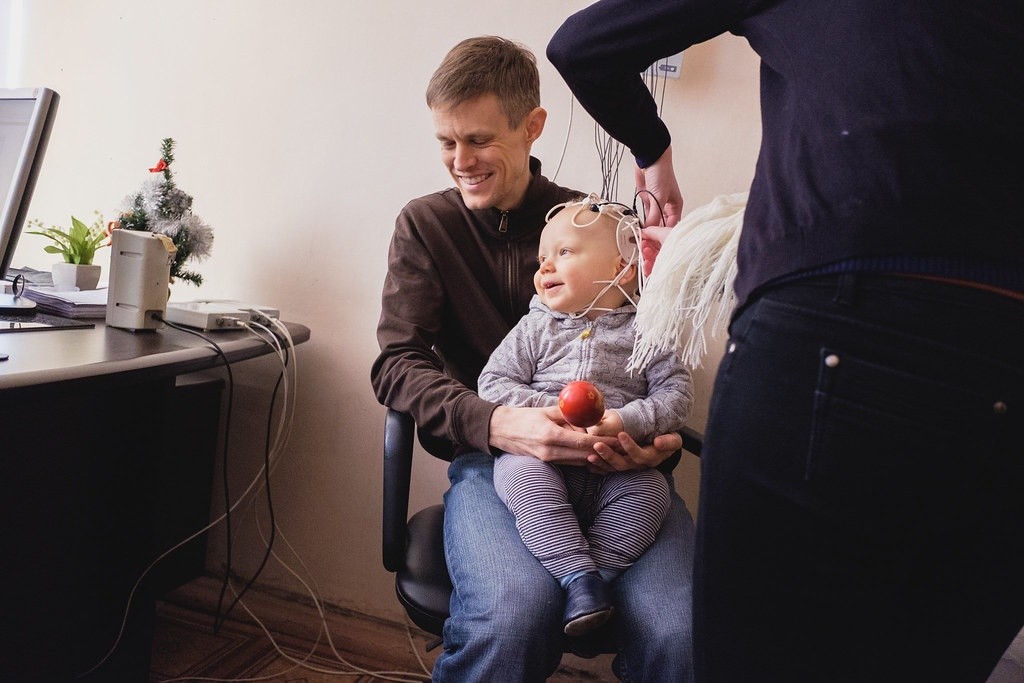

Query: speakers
<box><xmin>105</xmin><ymin>229</ymin><xmax>172</xmax><ymax>332</ymax></box>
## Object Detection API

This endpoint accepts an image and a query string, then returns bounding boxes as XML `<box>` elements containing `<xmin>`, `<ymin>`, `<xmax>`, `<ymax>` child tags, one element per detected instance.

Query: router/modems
<box><xmin>165</xmin><ymin>302</ymin><xmax>280</xmax><ymax>333</ymax></box>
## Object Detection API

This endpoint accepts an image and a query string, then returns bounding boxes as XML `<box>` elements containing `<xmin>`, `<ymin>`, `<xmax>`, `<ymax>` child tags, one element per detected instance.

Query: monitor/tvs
<box><xmin>0</xmin><ymin>86</ymin><xmax>60</xmax><ymax>280</ymax></box>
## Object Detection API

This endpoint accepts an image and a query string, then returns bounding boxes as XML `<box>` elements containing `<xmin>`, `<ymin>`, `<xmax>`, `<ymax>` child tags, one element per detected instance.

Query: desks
<box><xmin>0</xmin><ymin>279</ymin><xmax>313</xmax><ymax>681</ymax></box>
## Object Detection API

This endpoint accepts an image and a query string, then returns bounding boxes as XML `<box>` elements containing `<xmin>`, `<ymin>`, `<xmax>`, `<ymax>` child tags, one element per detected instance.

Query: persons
<box><xmin>476</xmin><ymin>197</ymin><xmax>695</xmax><ymax>640</ymax></box>
<box><xmin>546</xmin><ymin>0</ymin><xmax>1024</xmax><ymax>682</ymax></box>
<box><xmin>371</xmin><ymin>35</ymin><xmax>700</xmax><ymax>683</ymax></box>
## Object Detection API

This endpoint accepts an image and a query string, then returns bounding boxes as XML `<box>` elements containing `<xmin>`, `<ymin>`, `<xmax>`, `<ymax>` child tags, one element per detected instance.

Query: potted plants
<box><xmin>26</xmin><ymin>216</ymin><xmax>108</xmax><ymax>290</ymax></box>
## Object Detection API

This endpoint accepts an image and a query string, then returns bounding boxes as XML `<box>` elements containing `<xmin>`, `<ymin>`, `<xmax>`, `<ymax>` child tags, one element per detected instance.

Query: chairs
<box><xmin>378</xmin><ymin>407</ymin><xmax>709</xmax><ymax>652</ymax></box>
